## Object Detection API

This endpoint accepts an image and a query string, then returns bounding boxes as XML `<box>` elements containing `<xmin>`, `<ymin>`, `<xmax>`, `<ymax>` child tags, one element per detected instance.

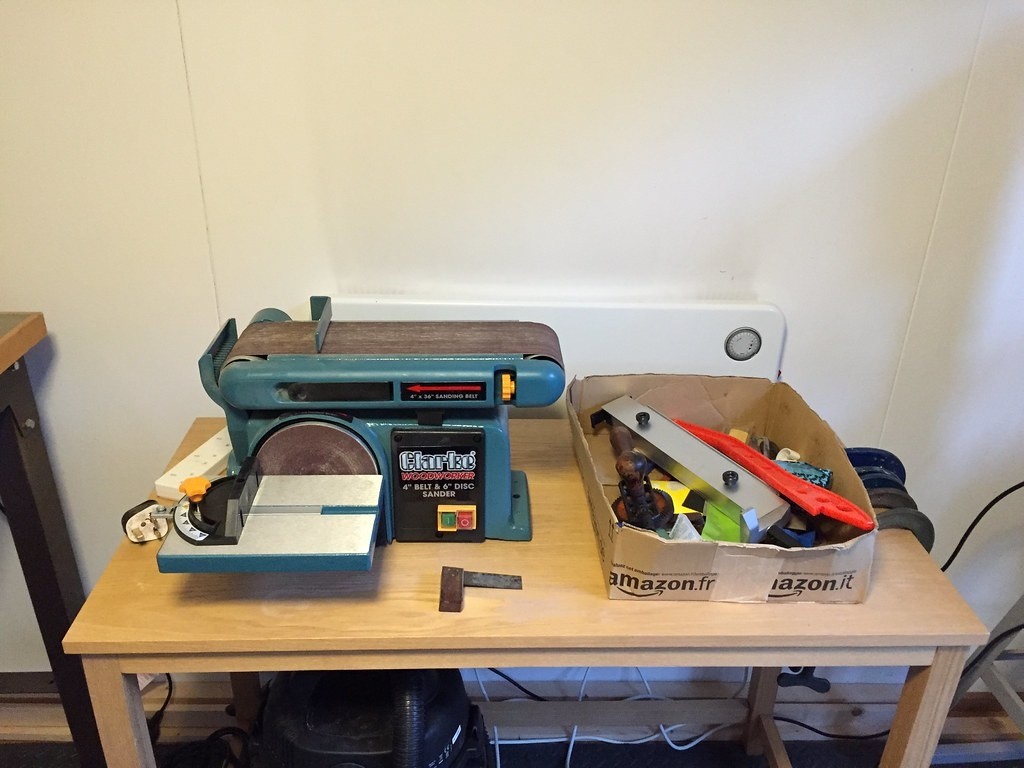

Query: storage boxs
<box><xmin>564</xmin><ymin>372</ymin><xmax>879</xmax><ymax>603</ymax></box>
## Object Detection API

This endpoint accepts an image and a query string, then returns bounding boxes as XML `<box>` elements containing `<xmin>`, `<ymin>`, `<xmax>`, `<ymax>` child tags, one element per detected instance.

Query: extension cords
<box><xmin>154</xmin><ymin>425</ymin><xmax>234</xmax><ymax>502</ymax></box>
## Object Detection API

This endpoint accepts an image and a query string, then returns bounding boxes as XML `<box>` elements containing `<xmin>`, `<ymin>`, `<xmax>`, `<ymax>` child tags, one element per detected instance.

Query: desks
<box><xmin>63</xmin><ymin>416</ymin><xmax>990</xmax><ymax>768</ymax></box>
<box><xmin>0</xmin><ymin>311</ymin><xmax>107</xmax><ymax>768</ymax></box>
